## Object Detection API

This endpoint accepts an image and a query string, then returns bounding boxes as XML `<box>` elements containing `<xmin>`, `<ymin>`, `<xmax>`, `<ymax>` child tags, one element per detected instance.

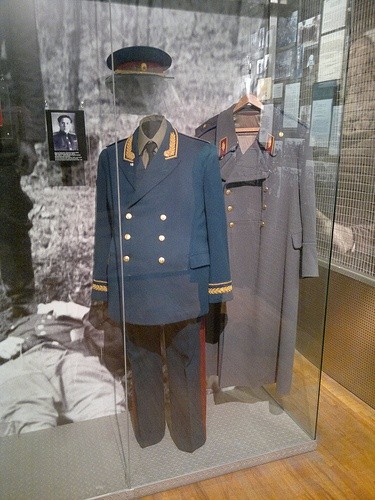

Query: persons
<box><xmin>0</xmin><ymin>1</ymin><xmax>47</xmax><ymax>316</ymax></box>
<box><xmin>53</xmin><ymin>113</ymin><xmax>80</xmax><ymax>153</ymax></box>
<box><xmin>0</xmin><ymin>295</ymin><xmax>129</xmax><ymax>443</ymax></box>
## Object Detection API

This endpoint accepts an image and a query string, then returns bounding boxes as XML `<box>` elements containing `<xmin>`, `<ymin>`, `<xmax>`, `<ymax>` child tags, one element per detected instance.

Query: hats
<box><xmin>104</xmin><ymin>43</ymin><xmax>175</xmax><ymax>75</ymax></box>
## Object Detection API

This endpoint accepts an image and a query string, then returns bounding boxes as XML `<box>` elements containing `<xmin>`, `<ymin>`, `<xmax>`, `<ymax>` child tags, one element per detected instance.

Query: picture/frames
<box><xmin>45</xmin><ymin>108</ymin><xmax>88</xmax><ymax>162</ymax></box>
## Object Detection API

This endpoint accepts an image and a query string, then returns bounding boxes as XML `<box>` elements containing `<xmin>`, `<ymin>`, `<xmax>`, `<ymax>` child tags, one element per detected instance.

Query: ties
<box><xmin>143</xmin><ymin>141</ymin><xmax>157</xmax><ymax>174</ymax></box>
